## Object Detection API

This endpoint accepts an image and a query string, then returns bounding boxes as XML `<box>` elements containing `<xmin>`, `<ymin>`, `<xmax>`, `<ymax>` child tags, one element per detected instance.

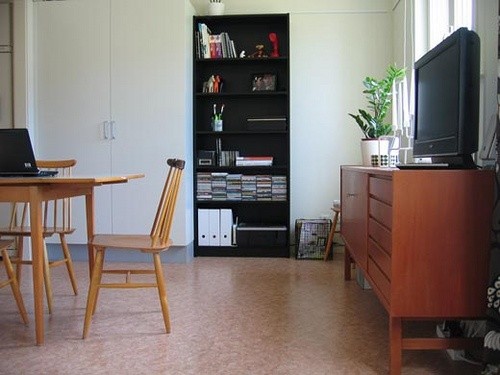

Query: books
<box><xmin>197</xmin><ymin>171</ymin><xmax>288</xmax><ymax>201</ymax></box>
<box><xmin>195</xmin><ymin>23</ymin><xmax>237</xmax><ymax>59</ymax></box>
<box><xmin>198</xmin><ymin>138</ymin><xmax>274</xmax><ymax>167</ymax></box>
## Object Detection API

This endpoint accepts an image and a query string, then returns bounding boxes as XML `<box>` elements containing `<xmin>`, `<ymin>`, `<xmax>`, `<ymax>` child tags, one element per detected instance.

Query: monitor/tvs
<box><xmin>413</xmin><ymin>27</ymin><xmax>480</xmax><ymax>158</ymax></box>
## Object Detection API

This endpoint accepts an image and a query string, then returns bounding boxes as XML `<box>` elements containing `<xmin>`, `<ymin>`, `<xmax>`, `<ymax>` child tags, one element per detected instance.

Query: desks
<box><xmin>0</xmin><ymin>173</ymin><xmax>144</xmax><ymax>346</ymax></box>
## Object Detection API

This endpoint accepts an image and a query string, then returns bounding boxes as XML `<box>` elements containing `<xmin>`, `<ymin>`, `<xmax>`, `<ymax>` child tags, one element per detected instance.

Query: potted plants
<box><xmin>348</xmin><ymin>62</ymin><xmax>404</xmax><ymax>167</ymax></box>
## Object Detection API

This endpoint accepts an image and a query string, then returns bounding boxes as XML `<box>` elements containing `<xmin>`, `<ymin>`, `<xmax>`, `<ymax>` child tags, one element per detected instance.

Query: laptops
<box><xmin>1</xmin><ymin>128</ymin><xmax>58</xmax><ymax>177</ymax></box>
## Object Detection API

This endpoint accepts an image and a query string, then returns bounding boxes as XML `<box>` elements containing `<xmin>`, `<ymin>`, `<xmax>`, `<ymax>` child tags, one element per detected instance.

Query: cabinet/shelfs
<box><xmin>33</xmin><ymin>2</ymin><xmax>190</xmax><ymax>247</ymax></box>
<box><xmin>369</xmin><ymin>175</ymin><xmax>394</xmax><ymax>309</ymax></box>
<box><xmin>193</xmin><ymin>16</ymin><xmax>290</xmax><ymax>259</ymax></box>
<box><xmin>341</xmin><ymin>166</ymin><xmax>368</xmax><ymax>271</ymax></box>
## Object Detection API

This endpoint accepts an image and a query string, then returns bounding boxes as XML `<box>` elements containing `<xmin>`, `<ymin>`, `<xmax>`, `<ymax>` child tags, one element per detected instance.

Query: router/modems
<box><xmin>396</xmin><ymin>163</ymin><xmax>449</xmax><ymax>170</ymax></box>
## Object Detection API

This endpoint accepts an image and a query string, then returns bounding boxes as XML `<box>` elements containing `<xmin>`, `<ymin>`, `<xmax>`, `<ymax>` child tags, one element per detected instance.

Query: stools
<box><xmin>324</xmin><ymin>207</ymin><xmax>341</xmax><ymax>261</ymax></box>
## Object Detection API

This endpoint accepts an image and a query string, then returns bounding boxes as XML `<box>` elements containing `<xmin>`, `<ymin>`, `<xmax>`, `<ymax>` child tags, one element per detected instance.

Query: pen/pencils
<box><xmin>213</xmin><ymin>103</ymin><xmax>218</xmax><ymax>120</ymax></box>
<box><xmin>218</xmin><ymin>103</ymin><xmax>225</xmax><ymax>120</ymax></box>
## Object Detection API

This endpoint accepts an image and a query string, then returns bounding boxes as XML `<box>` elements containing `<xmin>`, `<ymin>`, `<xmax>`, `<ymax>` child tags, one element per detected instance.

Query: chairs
<box><xmin>0</xmin><ymin>159</ymin><xmax>78</xmax><ymax>315</ymax></box>
<box><xmin>80</xmin><ymin>160</ymin><xmax>186</xmax><ymax>339</ymax></box>
<box><xmin>1</xmin><ymin>239</ymin><xmax>28</xmax><ymax>325</ymax></box>
